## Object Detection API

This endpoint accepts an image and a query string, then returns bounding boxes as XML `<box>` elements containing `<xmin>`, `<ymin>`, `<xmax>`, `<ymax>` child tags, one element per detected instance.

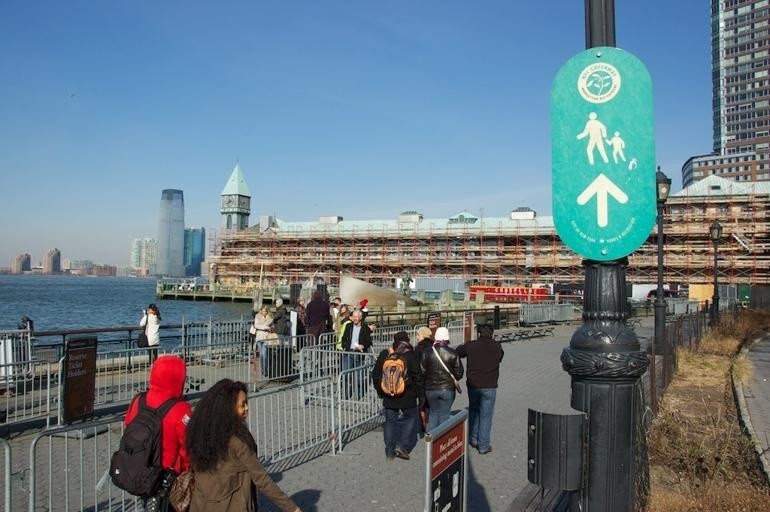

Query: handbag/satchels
<box><xmin>454</xmin><ymin>381</ymin><xmax>462</xmax><ymax>394</ymax></box>
<box><xmin>380</xmin><ymin>358</ymin><xmax>409</xmax><ymax>397</ymax></box>
<box><xmin>138</xmin><ymin>333</ymin><xmax>148</xmax><ymax>347</ymax></box>
<box><xmin>267</xmin><ymin>332</ymin><xmax>279</xmax><ymax>345</ymax></box>
<box><xmin>169</xmin><ymin>471</ymin><xmax>194</xmax><ymax>511</ymax></box>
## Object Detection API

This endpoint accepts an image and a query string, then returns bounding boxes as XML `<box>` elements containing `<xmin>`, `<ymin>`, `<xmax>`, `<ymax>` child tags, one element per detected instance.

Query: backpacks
<box><xmin>109</xmin><ymin>390</ymin><xmax>180</xmax><ymax>498</ymax></box>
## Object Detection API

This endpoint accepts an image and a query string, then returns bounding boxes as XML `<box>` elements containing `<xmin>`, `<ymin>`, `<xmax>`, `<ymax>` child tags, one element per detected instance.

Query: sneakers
<box><xmin>386</xmin><ymin>445</ymin><xmax>410</xmax><ymax>463</ymax></box>
<box><xmin>479</xmin><ymin>445</ymin><xmax>492</xmax><ymax>453</ymax></box>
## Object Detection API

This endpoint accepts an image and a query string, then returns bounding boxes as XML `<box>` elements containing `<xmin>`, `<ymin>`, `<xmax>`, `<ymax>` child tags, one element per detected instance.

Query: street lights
<box><xmin>653</xmin><ymin>165</ymin><xmax>672</xmax><ymax>348</ymax></box>
<box><xmin>707</xmin><ymin>219</ymin><xmax>724</xmax><ymax>327</ymax></box>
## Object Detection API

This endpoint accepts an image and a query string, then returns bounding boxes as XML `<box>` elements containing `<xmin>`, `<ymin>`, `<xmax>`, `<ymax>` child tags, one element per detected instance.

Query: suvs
<box><xmin>647</xmin><ymin>289</ymin><xmax>687</xmax><ymax>304</ymax></box>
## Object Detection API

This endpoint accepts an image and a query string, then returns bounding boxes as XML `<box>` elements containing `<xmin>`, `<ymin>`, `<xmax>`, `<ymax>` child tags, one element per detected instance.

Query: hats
<box><xmin>435</xmin><ymin>327</ymin><xmax>450</xmax><ymax>341</ymax></box>
<box><xmin>417</xmin><ymin>327</ymin><xmax>432</xmax><ymax>338</ymax></box>
<box><xmin>394</xmin><ymin>332</ymin><xmax>410</xmax><ymax>342</ymax></box>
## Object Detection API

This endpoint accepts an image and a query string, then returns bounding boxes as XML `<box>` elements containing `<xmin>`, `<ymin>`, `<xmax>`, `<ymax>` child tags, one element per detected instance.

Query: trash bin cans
<box><xmin>261</xmin><ymin>344</ymin><xmax>292</xmax><ymax>383</ymax></box>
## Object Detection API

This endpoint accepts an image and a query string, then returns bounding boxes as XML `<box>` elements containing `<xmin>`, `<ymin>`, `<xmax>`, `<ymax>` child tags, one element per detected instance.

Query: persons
<box><xmin>180</xmin><ymin>281</ymin><xmax>195</xmax><ymax>290</ymax></box>
<box><xmin>372</xmin><ymin>326</ymin><xmax>464</xmax><ymax>461</ymax></box>
<box><xmin>457</xmin><ymin>324</ymin><xmax>504</xmax><ymax>453</ymax></box>
<box><xmin>140</xmin><ymin>303</ymin><xmax>161</xmax><ymax>365</ymax></box>
<box><xmin>576</xmin><ymin>112</ymin><xmax>608</xmax><ymax>165</ymax></box>
<box><xmin>607</xmin><ymin>131</ymin><xmax>628</xmax><ymax>163</ymax></box>
<box><xmin>254</xmin><ymin>292</ymin><xmax>372</xmax><ymax>402</ymax></box>
<box><xmin>186</xmin><ymin>378</ymin><xmax>301</xmax><ymax>511</ymax></box>
<box><xmin>126</xmin><ymin>356</ymin><xmax>193</xmax><ymax>511</ymax></box>
